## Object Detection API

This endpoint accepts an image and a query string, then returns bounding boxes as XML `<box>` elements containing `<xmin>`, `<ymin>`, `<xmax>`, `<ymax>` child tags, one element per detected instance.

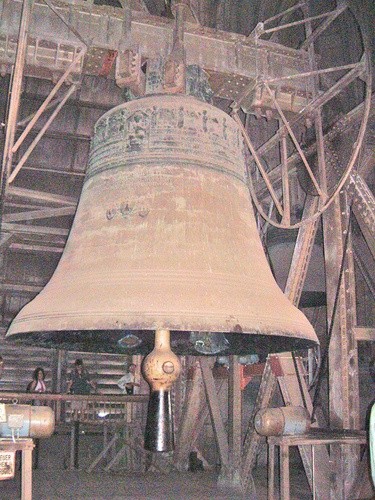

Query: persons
<box><xmin>117</xmin><ymin>362</ymin><xmax>143</xmax><ymax>396</ymax></box>
<box><xmin>66</xmin><ymin>359</ymin><xmax>102</xmax><ymax>423</ymax></box>
<box><xmin>26</xmin><ymin>367</ymin><xmax>51</xmax><ymax>408</ymax></box>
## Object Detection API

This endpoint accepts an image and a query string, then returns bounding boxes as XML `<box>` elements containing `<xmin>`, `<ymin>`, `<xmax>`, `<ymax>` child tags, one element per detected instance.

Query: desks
<box><xmin>267</xmin><ymin>429</ymin><xmax>369</xmax><ymax>500</ymax></box>
<box><xmin>0</xmin><ymin>438</ymin><xmax>36</xmax><ymax>500</ymax></box>
